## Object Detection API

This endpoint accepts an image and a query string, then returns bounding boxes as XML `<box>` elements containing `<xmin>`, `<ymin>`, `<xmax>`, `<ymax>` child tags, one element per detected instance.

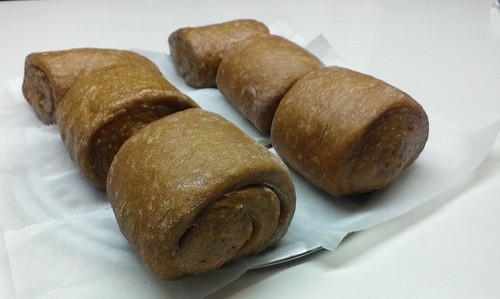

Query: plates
<box><xmin>21</xmin><ymin>0</ymin><xmax>500</xmax><ymax>269</ymax></box>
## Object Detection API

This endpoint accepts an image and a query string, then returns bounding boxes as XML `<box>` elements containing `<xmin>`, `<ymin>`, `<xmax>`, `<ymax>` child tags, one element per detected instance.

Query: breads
<box><xmin>107</xmin><ymin>108</ymin><xmax>297</xmax><ymax>280</ymax></box>
<box><xmin>22</xmin><ymin>48</ymin><xmax>163</xmax><ymax>126</ymax></box>
<box><xmin>54</xmin><ymin>61</ymin><xmax>200</xmax><ymax>192</ymax></box>
<box><xmin>216</xmin><ymin>34</ymin><xmax>326</xmax><ymax>135</ymax></box>
<box><xmin>168</xmin><ymin>19</ymin><xmax>270</xmax><ymax>88</ymax></box>
<box><xmin>271</xmin><ymin>66</ymin><xmax>430</xmax><ymax>195</ymax></box>
